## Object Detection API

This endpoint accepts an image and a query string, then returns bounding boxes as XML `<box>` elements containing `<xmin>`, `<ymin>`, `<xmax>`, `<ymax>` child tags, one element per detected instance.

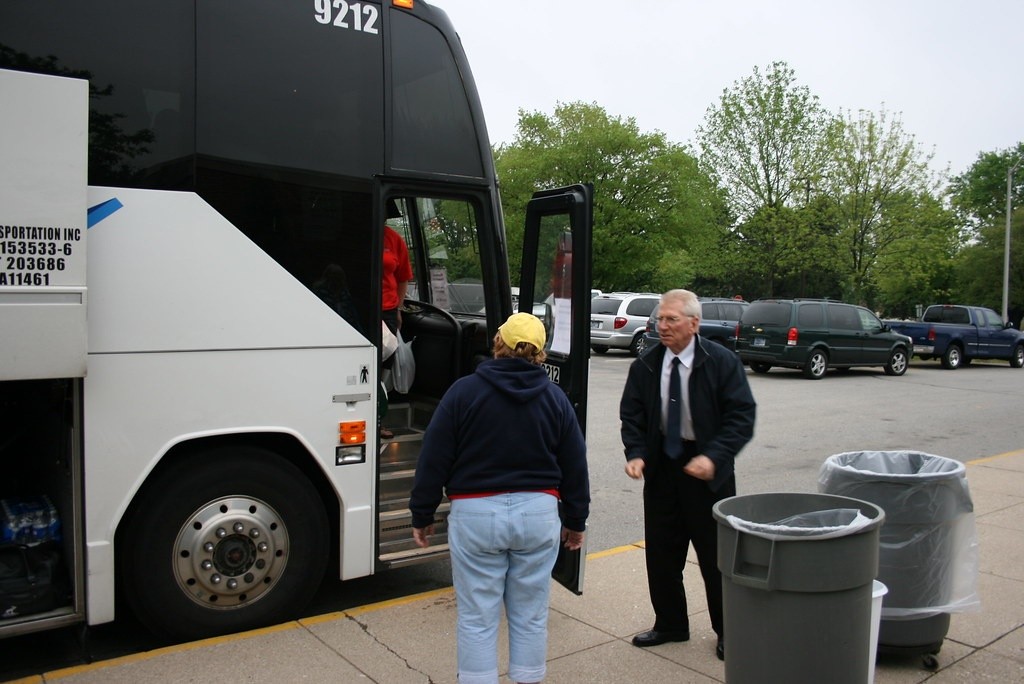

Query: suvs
<box><xmin>733</xmin><ymin>298</ymin><xmax>913</xmax><ymax>381</ymax></box>
<box><xmin>641</xmin><ymin>296</ymin><xmax>751</xmax><ymax>356</ymax></box>
<box><xmin>587</xmin><ymin>292</ymin><xmax>663</xmax><ymax>358</ymax></box>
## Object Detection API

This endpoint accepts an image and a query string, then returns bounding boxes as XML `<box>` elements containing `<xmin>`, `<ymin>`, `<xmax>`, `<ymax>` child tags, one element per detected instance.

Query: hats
<box><xmin>498</xmin><ymin>311</ymin><xmax>546</xmax><ymax>356</ymax></box>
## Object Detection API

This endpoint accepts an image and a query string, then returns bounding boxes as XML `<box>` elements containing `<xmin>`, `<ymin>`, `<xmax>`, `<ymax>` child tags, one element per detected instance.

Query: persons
<box><xmin>620</xmin><ymin>288</ymin><xmax>757</xmax><ymax>659</ymax></box>
<box><xmin>408</xmin><ymin>312</ymin><xmax>589</xmax><ymax>684</ymax></box>
<box><xmin>382</xmin><ymin>208</ymin><xmax>414</xmax><ymax>439</ymax></box>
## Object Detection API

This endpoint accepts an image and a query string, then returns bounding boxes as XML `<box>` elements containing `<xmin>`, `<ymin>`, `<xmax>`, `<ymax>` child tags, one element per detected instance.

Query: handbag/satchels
<box><xmin>391</xmin><ymin>325</ymin><xmax>416</xmax><ymax>395</ymax></box>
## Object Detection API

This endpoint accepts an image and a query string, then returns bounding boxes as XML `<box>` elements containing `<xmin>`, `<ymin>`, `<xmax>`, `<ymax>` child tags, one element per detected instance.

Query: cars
<box><xmin>467</xmin><ymin>288</ymin><xmax>603</xmax><ymax>326</ymax></box>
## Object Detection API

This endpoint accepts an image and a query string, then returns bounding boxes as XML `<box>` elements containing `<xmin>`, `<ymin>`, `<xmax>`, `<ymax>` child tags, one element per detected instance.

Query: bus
<box><xmin>1</xmin><ymin>0</ymin><xmax>596</xmax><ymax>652</ymax></box>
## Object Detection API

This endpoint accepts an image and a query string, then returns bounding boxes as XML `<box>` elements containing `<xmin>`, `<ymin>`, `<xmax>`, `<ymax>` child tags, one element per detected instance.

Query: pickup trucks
<box><xmin>879</xmin><ymin>305</ymin><xmax>1024</xmax><ymax>371</ymax></box>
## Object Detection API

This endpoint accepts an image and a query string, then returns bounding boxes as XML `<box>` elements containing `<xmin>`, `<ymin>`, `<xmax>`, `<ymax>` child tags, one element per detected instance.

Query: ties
<box><xmin>665</xmin><ymin>358</ymin><xmax>682</xmax><ymax>459</ymax></box>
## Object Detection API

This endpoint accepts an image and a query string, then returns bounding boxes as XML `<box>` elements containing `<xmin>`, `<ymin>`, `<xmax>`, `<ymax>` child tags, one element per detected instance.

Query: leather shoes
<box><xmin>633</xmin><ymin>629</ymin><xmax>691</xmax><ymax>647</ymax></box>
<box><xmin>716</xmin><ymin>635</ymin><xmax>726</xmax><ymax>661</ymax></box>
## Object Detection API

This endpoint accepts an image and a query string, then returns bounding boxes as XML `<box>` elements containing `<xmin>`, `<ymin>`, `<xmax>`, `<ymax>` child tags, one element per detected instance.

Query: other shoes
<box><xmin>379</xmin><ymin>427</ymin><xmax>393</xmax><ymax>439</ymax></box>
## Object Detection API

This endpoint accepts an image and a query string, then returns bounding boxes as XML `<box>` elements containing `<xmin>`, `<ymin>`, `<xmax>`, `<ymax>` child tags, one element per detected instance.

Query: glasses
<box><xmin>652</xmin><ymin>313</ymin><xmax>694</xmax><ymax>326</ymax></box>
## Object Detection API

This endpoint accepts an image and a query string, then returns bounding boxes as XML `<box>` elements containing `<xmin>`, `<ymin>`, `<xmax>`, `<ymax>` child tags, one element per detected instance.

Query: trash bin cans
<box><xmin>868</xmin><ymin>579</ymin><xmax>889</xmax><ymax>684</ymax></box>
<box><xmin>816</xmin><ymin>450</ymin><xmax>975</xmax><ymax>674</ymax></box>
<box><xmin>711</xmin><ymin>491</ymin><xmax>887</xmax><ymax>684</ymax></box>
